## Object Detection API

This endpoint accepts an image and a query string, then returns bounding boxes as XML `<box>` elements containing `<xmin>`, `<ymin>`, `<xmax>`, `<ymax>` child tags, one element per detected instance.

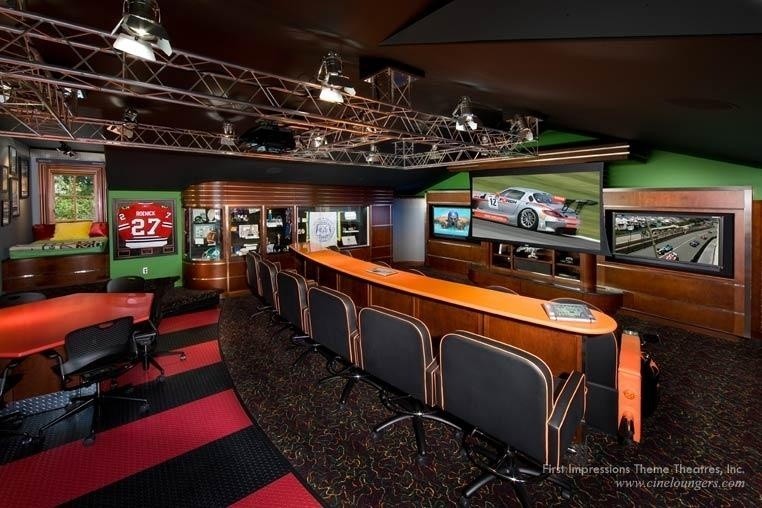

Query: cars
<box><xmin>655</xmin><ymin>244</ymin><xmax>673</xmax><ymax>255</ymax></box>
<box><xmin>472</xmin><ymin>187</ymin><xmax>598</xmax><ymax>234</ymax></box>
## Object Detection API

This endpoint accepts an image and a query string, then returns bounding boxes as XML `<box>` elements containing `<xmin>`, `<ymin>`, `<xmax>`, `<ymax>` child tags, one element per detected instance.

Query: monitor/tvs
<box><xmin>605</xmin><ymin>211</ymin><xmax>734</xmax><ymax>279</ymax></box>
<box><xmin>429</xmin><ymin>204</ymin><xmax>481</xmax><ymax>246</ymax></box>
<box><xmin>470</xmin><ymin>161</ymin><xmax>609</xmax><ymax>255</ymax></box>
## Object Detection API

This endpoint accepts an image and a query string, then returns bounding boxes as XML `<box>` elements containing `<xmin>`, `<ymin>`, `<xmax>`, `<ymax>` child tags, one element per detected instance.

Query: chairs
<box><xmin>241</xmin><ymin>244</ymin><xmax>663</xmax><ymax>507</ymax></box>
<box><xmin>0</xmin><ymin>275</ymin><xmax>187</xmax><ymax>447</ymax></box>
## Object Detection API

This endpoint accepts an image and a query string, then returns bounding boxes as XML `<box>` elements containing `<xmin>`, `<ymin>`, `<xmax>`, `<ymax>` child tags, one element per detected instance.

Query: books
<box><xmin>541</xmin><ymin>301</ymin><xmax>597</xmax><ymax>324</ymax></box>
<box><xmin>366</xmin><ymin>266</ymin><xmax>399</xmax><ymax>278</ymax></box>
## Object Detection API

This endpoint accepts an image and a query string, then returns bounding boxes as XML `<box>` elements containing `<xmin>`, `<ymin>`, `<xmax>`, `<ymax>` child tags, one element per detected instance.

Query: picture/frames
<box><xmin>0</xmin><ymin>141</ymin><xmax>32</xmax><ymax>228</ymax></box>
<box><xmin>112</xmin><ymin>198</ymin><xmax>179</xmax><ymax>260</ymax></box>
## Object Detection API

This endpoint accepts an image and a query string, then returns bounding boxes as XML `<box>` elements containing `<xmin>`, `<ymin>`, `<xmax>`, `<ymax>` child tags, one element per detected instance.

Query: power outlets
<box><xmin>143</xmin><ymin>266</ymin><xmax>149</xmax><ymax>275</ymax></box>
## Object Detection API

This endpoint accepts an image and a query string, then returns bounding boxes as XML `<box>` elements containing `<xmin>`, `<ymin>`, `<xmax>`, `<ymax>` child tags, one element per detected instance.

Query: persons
<box><xmin>434</xmin><ymin>210</ymin><xmax>469</xmax><ymax>230</ymax></box>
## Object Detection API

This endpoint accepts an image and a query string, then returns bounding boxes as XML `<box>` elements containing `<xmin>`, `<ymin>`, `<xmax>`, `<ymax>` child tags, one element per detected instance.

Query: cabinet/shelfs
<box><xmin>183</xmin><ymin>205</ymin><xmax>371</xmax><ymax>292</ymax></box>
<box><xmin>0</xmin><ymin>254</ymin><xmax>111</xmax><ymax>292</ymax></box>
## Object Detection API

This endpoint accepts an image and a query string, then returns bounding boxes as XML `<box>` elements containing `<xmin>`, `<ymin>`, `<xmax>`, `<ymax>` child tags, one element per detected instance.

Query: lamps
<box><xmin>114</xmin><ymin>102</ymin><xmax>383</xmax><ymax>163</ymax></box>
<box><xmin>109</xmin><ymin>0</ymin><xmax>538</xmax><ymax>145</ymax></box>
<box><xmin>54</xmin><ymin>140</ymin><xmax>77</xmax><ymax>158</ymax></box>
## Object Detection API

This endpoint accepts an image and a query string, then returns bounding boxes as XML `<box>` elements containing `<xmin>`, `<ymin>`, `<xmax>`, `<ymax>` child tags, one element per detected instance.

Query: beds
<box><xmin>8</xmin><ymin>219</ymin><xmax>108</xmax><ymax>258</ymax></box>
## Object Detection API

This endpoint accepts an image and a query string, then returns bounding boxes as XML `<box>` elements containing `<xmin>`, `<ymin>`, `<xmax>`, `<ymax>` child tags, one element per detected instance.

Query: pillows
<box><xmin>50</xmin><ymin>221</ymin><xmax>93</xmax><ymax>241</ymax></box>
